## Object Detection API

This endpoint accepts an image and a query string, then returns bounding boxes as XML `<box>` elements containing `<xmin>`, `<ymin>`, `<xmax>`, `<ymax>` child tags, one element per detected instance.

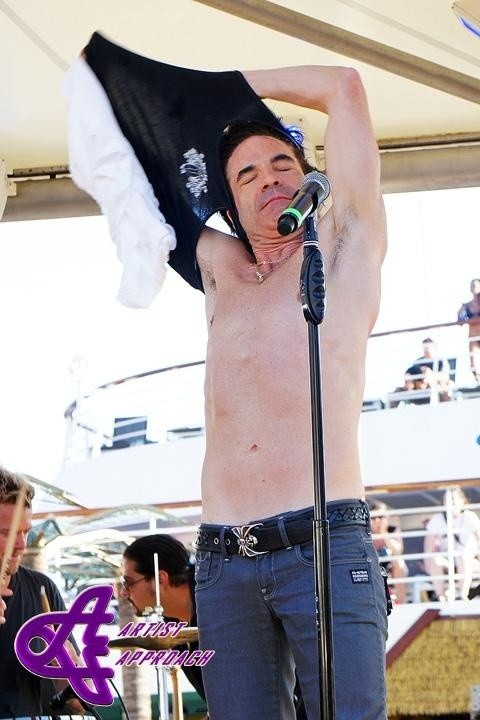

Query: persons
<box><xmin>391</xmin><ymin>338</ymin><xmax>454</xmax><ymax>410</ymax></box>
<box><xmin>366</xmin><ymin>500</ymin><xmax>408</xmax><ymax>604</ymax></box>
<box><xmin>2</xmin><ymin>470</ymin><xmax>95</xmax><ymax>720</ymax></box>
<box><xmin>68</xmin><ymin>31</ymin><xmax>390</xmax><ymax>720</ymax></box>
<box><xmin>421</xmin><ymin>485</ymin><xmax>479</xmax><ymax>601</ymax></box>
<box><xmin>118</xmin><ymin>531</ymin><xmax>306</xmax><ymax>720</ymax></box>
<box><xmin>457</xmin><ymin>278</ymin><xmax>479</xmax><ymax>396</ymax></box>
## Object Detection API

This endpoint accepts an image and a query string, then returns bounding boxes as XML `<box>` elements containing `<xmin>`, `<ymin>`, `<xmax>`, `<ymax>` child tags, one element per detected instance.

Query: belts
<box><xmin>194</xmin><ymin>498</ymin><xmax>374</xmax><ymax>556</ymax></box>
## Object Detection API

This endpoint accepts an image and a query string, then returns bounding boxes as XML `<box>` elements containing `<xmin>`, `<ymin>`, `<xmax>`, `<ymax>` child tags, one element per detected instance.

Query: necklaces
<box><xmin>251</xmin><ymin>263</ymin><xmax>285</xmax><ymax>284</ymax></box>
<box><xmin>255</xmin><ymin>257</ymin><xmax>290</xmax><ymax>265</ymax></box>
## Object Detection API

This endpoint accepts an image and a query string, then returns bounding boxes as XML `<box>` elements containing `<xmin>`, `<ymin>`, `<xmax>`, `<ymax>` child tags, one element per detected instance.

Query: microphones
<box><xmin>277</xmin><ymin>170</ymin><xmax>332</xmax><ymax>236</ymax></box>
<box><xmin>46</xmin><ymin>682</ymin><xmax>75</xmax><ymax>712</ymax></box>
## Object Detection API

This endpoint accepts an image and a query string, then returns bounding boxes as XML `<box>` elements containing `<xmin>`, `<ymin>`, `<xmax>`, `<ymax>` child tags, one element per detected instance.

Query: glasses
<box><xmin>116</xmin><ymin>575</ymin><xmax>145</xmax><ymax>590</ymax></box>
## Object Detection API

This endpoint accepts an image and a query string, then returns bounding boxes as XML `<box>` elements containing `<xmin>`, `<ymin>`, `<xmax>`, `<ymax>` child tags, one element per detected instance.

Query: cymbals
<box><xmin>106</xmin><ymin>627</ymin><xmax>199</xmax><ymax>651</ymax></box>
